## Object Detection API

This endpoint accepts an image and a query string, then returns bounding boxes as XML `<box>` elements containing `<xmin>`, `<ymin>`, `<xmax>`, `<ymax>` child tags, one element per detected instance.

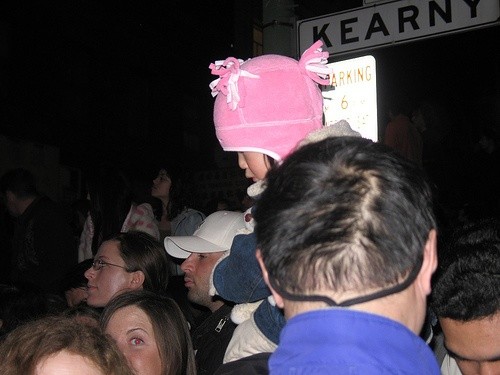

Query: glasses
<box><xmin>92</xmin><ymin>259</ymin><xmax>127</xmax><ymax>271</ymax></box>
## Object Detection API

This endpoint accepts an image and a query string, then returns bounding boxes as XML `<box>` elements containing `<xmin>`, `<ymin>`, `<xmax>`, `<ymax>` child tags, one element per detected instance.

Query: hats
<box><xmin>208</xmin><ymin>39</ymin><xmax>335</xmax><ymax>168</ymax></box>
<box><xmin>164</xmin><ymin>211</ymin><xmax>255</xmax><ymax>259</ymax></box>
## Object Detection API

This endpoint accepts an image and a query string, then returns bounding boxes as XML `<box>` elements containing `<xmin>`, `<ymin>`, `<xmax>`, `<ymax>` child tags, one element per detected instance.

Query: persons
<box><xmin>215</xmin><ymin>136</ymin><xmax>442</xmax><ymax>375</ymax></box>
<box><xmin>209</xmin><ymin>41</ymin><xmax>363</xmax><ymax>363</ymax></box>
<box><xmin>382</xmin><ymin>102</ymin><xmax>500</xmax><ymax>375</ymax></box>
<box><xmin>0</xmin><ymin>161</ymin><xmax>248</xmax><ymax>375</ymax></box>
